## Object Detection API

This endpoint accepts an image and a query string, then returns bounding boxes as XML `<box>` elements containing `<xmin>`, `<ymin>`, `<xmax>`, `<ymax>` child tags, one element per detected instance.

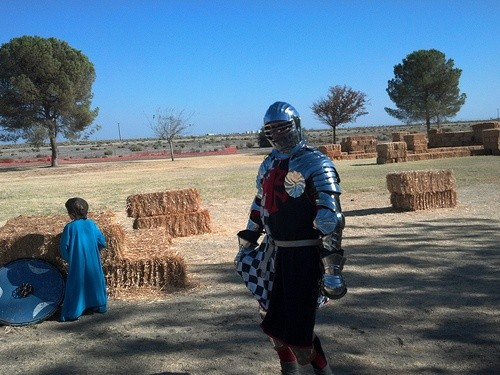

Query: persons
<box><xmin>236</xmin><ymin>101</ymin><xmax>349</xmax><ymax>375</ymax></box>
<box><xmin>59</xmin><ymin>197</ymin><xmax>109</xmax><ymax>321</ymax></box>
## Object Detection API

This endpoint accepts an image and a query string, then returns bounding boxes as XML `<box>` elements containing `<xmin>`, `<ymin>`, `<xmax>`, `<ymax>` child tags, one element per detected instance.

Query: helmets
<box><xmin>262</xmin><ymin>102</ymin><xmax>302</xmax><ymax>153</ymax></box>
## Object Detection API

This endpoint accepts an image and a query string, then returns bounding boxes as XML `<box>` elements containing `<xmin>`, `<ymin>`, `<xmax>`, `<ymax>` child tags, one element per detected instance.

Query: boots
<box><xmin>277</xmin><ymin>358</ymin><xmax>301</xmax><ymax>375</ymax></box>
<box><xmin>313</xmin><ymin>363</ymin><xmax>335</xmax><ymax>375</ymax></box>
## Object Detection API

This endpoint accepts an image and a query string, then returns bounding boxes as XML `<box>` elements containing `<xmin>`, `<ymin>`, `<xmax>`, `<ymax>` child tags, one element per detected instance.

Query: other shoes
<box><xmin>92</xmin><ymin>303</ymin><xmax>107</xmax><ymax>314</ymax></box>
